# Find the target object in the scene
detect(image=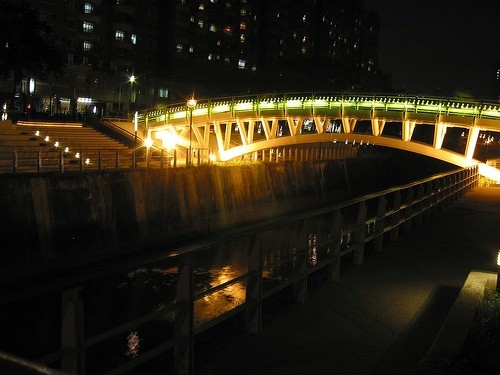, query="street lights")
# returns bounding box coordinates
[134,111,138,170]
[186,92,197,167]
